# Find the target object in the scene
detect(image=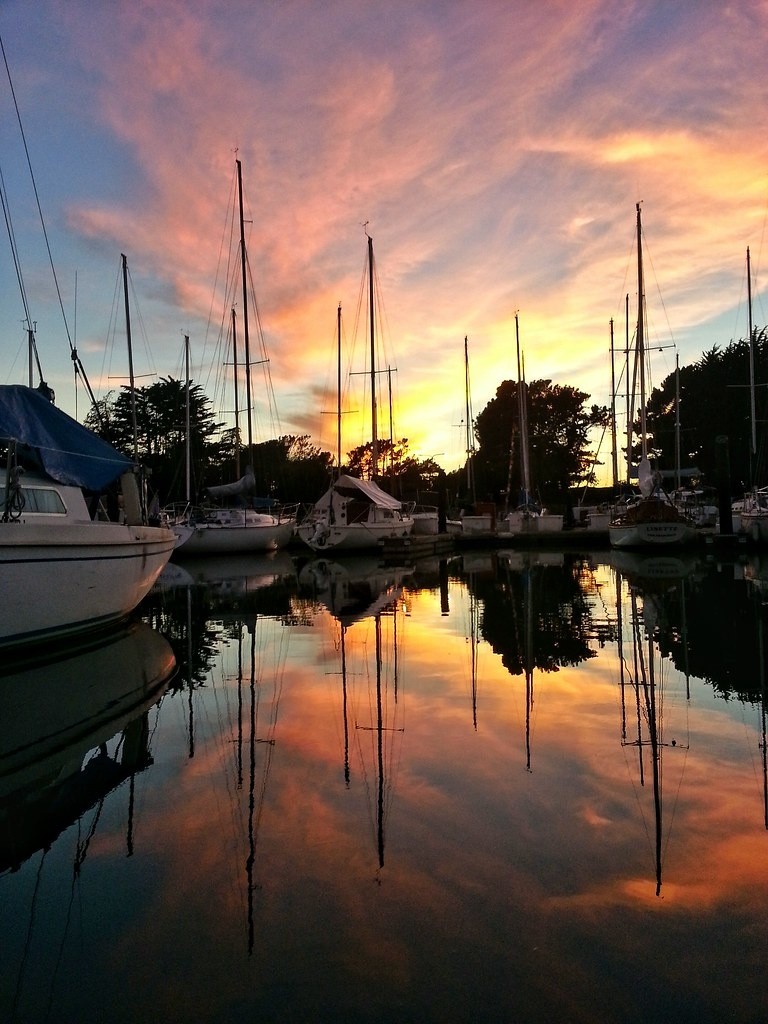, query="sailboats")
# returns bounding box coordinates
[0,162,767,644]
